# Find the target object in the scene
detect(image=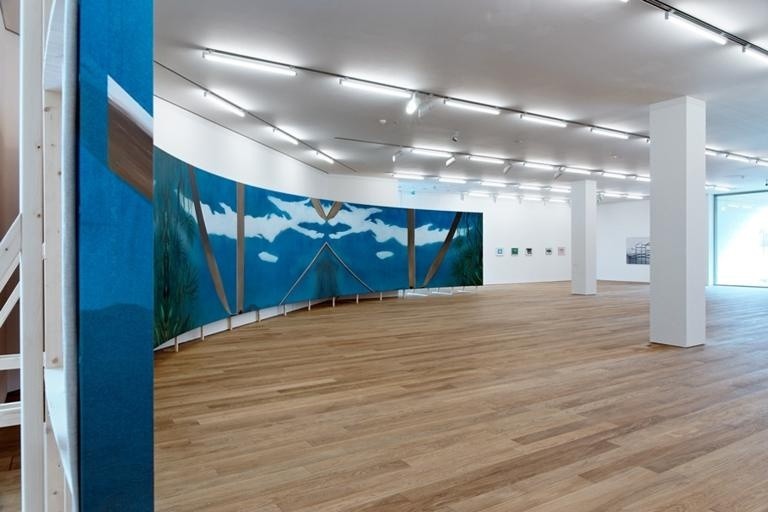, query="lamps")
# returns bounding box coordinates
[199,3,768,201]
